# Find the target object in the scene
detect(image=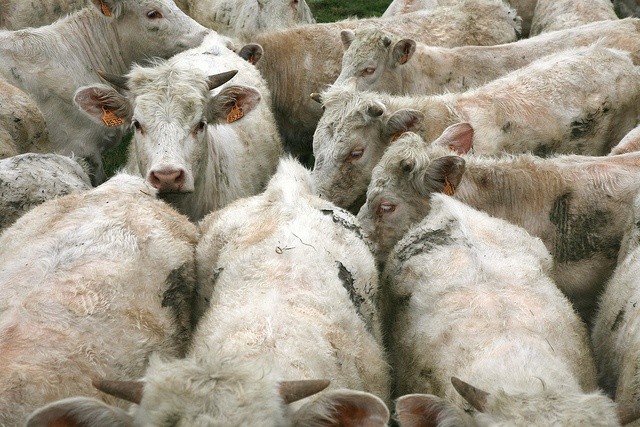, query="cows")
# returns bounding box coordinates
[333,16,640,97]
[173,0,314,41]
[309,45,640,206]
[531,1,619,35]
[0,154,84,232]
[0,0,237,187]
[74,28,281,220]
[381,193,640,427]
[0,169,199,427]
[590,192,640,427]
[90,155,391,427]
[255,0,522,171]
[0,0,85,35]
[356,121,640,318]
